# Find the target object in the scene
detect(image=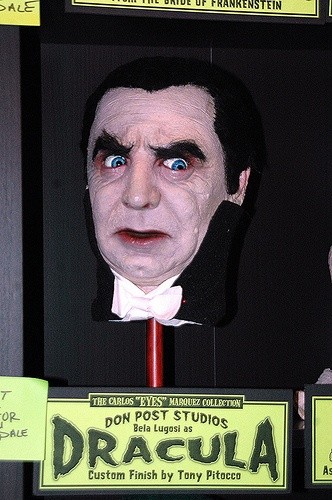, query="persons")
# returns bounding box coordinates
[80,57,265,326]
